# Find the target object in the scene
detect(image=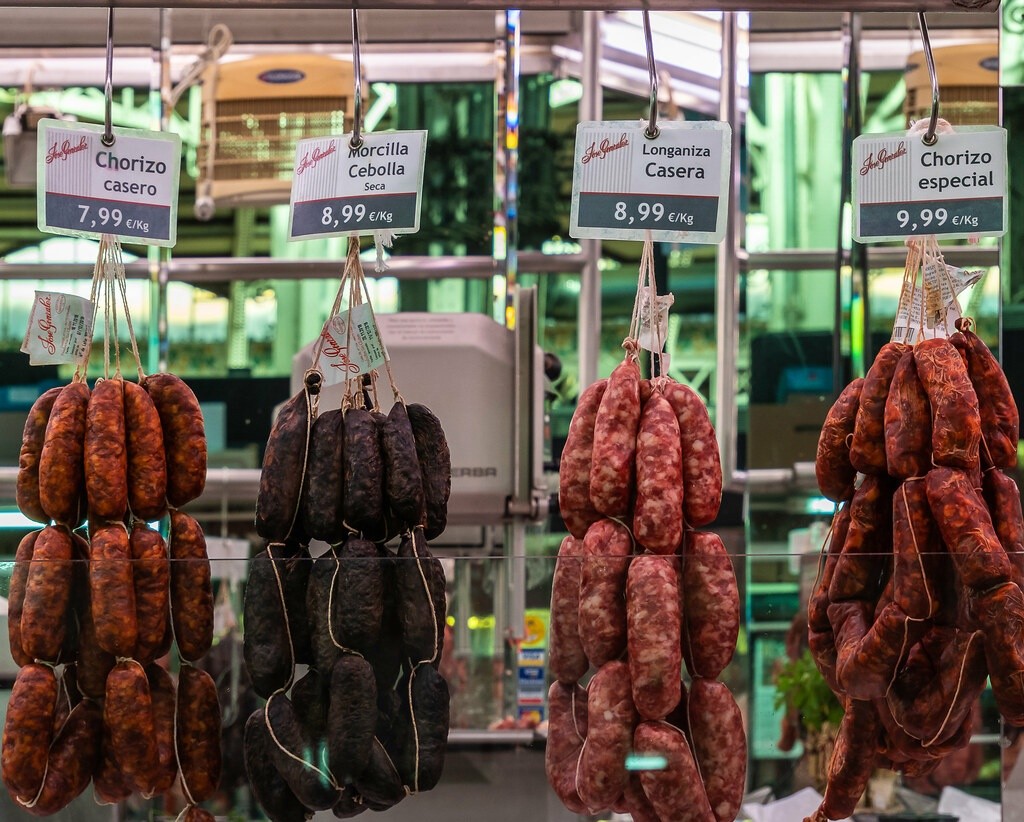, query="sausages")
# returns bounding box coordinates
[0,371,451,822]
[548,329,1024,822]
